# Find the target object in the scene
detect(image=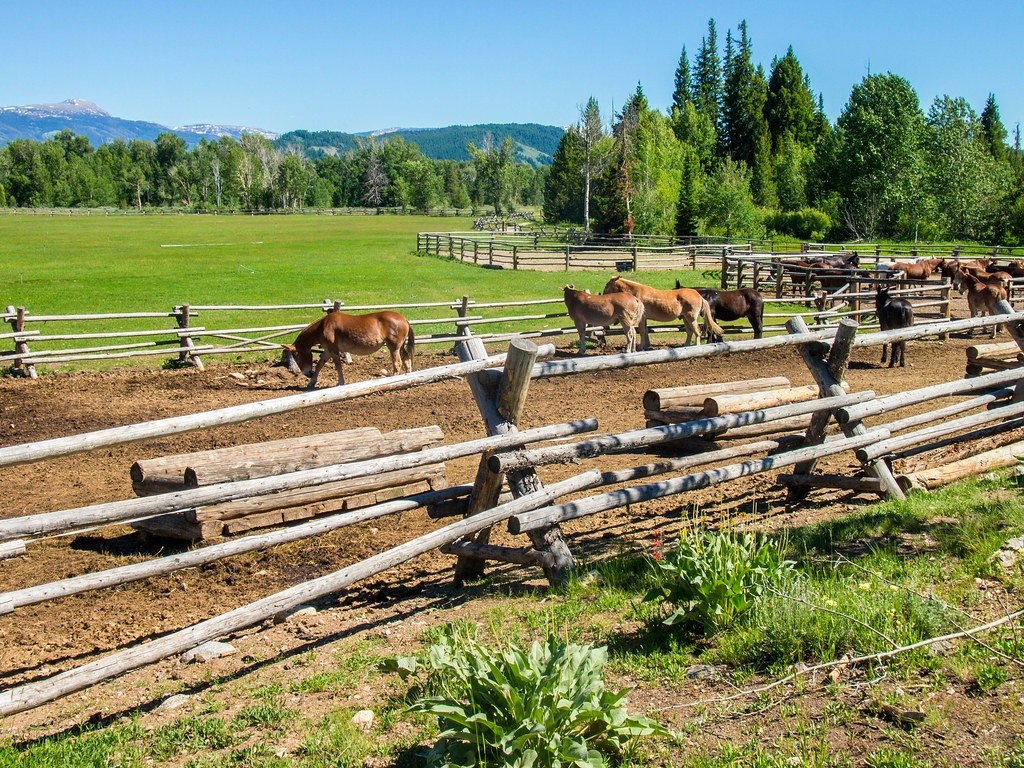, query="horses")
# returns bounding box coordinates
[561,284,645,357]
[281,311,415,391]
[770,251,872,312]
[603,275,725,352]
[875,285,914,369]
[875,255,1024,339]
[672,278,764,344]
